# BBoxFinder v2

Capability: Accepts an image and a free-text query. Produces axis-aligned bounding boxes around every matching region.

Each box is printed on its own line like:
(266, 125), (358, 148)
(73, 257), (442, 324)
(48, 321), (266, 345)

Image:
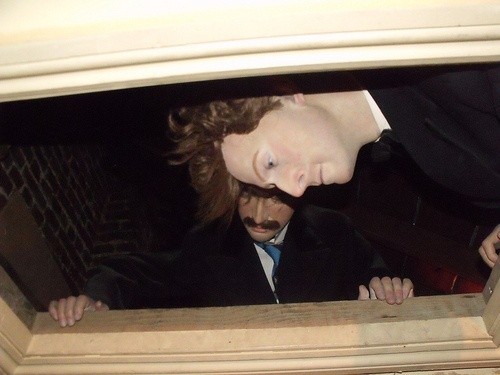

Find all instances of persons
(151, 72), (498, 272)
(47, 165), (416, 328)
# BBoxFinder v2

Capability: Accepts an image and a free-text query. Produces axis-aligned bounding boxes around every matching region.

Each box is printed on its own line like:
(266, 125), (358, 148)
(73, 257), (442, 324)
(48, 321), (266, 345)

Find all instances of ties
(257, 242), (282, 297)
